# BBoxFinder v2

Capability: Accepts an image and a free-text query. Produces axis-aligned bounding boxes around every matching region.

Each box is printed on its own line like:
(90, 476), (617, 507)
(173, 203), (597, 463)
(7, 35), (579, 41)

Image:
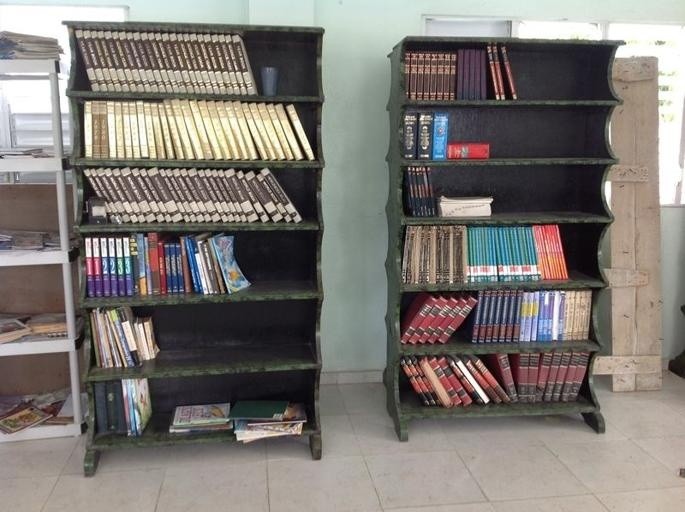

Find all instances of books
(84, 98), (315, 162)
(85, 232), (250, 296)
(402, 225), (593, 408)
(407, 165), (495, 219)
(75, 30), (259, 96)
(1, 28), (66, 62)
(449, 142), (491, 158)
(403, 113), (417, 160)
(433, 111), (448, 161)
(83, 167), (303, 224)
(94, 377), (310, 445)
(0, 144), (82, 439)
(416, 111), (433, 161)
(403, 44), (520, 102)
(88, 304), (160, 369)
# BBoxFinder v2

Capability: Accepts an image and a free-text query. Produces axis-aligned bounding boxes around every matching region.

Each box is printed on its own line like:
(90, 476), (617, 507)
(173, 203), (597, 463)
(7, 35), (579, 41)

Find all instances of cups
(260, 64), (280, 97)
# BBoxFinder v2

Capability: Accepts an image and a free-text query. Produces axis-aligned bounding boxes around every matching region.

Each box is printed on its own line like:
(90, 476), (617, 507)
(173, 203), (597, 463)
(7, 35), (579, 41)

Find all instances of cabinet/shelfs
(1, 58), (91, 443)
(383, 39), (628, 441)
(61, 20), (325, 477)
